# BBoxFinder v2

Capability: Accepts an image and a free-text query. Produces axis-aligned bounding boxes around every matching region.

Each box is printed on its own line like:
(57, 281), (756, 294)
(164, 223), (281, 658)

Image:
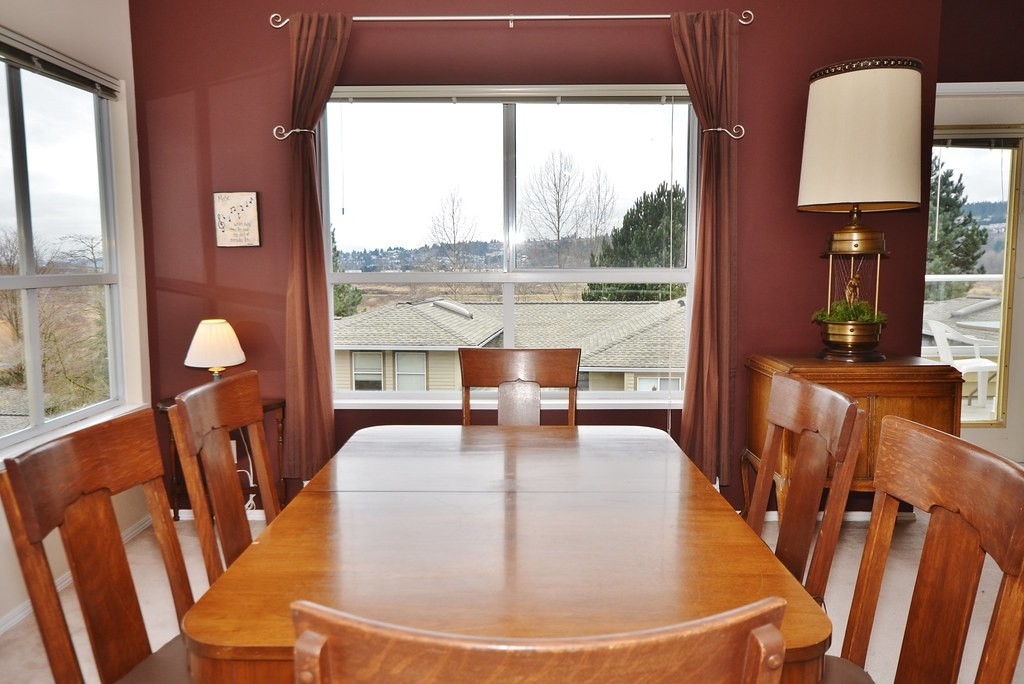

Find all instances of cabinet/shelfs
(738, 351), (964, 520)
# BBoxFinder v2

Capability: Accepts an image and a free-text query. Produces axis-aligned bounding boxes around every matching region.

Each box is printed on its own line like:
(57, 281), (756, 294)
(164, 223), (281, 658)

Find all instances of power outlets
(228, 440), (237, 465)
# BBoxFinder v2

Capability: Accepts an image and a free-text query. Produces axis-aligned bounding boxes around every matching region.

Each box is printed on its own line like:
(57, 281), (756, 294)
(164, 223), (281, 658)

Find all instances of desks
(157, 398), (286, 521)
(182, 424), (832, 684)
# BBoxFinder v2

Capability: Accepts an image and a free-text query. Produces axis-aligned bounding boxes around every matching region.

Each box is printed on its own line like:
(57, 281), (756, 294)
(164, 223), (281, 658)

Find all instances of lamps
(797, 57), (923, 363)
(183, 319), (247, 381)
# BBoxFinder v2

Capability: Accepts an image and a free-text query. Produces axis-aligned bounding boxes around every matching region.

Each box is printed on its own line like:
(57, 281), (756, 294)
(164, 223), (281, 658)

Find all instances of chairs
(927, 320), (999, 405)
(290, 596), (788, 684)
(822, 415), (1024, 684)
(0, 409), (195, 684)
(459, 347), (581, 425)
(746, 372), (867, 607)
(175, 371), (282, 588)
